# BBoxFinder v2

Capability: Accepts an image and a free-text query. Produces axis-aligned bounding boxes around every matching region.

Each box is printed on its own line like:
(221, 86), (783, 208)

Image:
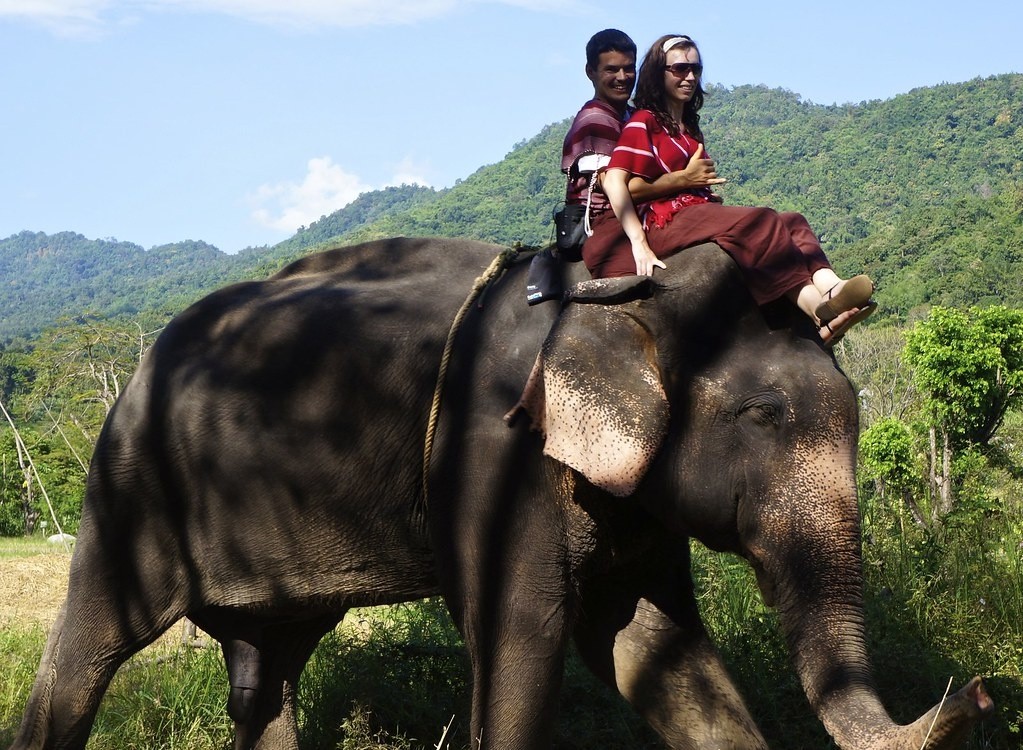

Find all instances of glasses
(665, 63), (703, 77)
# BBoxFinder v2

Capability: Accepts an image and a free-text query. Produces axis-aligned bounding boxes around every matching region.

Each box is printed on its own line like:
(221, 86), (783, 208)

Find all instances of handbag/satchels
(551, 204), (587, 262)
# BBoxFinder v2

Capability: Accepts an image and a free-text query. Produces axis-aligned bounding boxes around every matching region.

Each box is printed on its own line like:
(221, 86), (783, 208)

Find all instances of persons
(560, 29), (878, 347)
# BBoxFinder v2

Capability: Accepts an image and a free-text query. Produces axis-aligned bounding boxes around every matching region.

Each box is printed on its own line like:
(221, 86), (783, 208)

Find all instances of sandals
(815, 274), (872, 320)
(817, 303), (877, 349)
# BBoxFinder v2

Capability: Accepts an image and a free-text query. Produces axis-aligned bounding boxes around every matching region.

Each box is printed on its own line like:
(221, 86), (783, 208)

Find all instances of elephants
(12, 239), (994, 750)
